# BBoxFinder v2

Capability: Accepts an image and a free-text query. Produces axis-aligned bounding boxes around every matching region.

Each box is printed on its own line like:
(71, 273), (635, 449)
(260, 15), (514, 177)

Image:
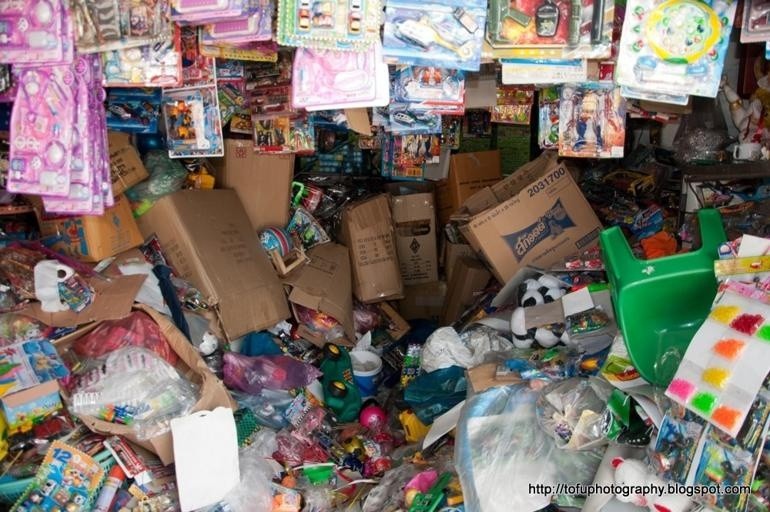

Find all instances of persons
(0, 1), (770, 512)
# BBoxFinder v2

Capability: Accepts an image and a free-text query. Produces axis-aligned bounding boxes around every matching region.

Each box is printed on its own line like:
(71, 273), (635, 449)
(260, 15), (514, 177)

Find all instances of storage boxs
(25, 127), (606, 467)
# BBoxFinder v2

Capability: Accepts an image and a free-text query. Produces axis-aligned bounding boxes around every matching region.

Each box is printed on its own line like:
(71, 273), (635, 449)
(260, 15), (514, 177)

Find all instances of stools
(598, 207), (732, 389)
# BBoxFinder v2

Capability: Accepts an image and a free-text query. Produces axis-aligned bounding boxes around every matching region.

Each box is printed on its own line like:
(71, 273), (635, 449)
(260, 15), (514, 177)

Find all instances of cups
(732, 142), (761, 162)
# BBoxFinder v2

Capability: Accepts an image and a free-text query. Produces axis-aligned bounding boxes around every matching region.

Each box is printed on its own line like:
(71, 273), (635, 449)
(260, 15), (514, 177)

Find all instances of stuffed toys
(0, 1), (770, 512)
